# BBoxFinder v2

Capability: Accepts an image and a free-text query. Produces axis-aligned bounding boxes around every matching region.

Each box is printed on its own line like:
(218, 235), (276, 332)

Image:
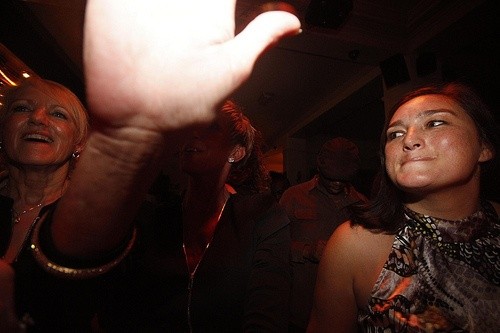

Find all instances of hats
(317, 137), (356, 182)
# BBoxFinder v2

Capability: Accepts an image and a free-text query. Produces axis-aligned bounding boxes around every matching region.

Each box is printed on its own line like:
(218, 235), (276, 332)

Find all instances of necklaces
(11, 203), (44, 223)
(183, 239), (208, 247)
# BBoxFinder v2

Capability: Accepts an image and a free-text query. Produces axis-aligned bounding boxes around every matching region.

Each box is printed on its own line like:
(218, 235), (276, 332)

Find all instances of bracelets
(30, 211), (138, 279)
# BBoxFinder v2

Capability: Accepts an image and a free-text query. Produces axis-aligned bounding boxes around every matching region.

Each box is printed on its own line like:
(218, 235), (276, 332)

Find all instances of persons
(0, 0), (500, 333)
